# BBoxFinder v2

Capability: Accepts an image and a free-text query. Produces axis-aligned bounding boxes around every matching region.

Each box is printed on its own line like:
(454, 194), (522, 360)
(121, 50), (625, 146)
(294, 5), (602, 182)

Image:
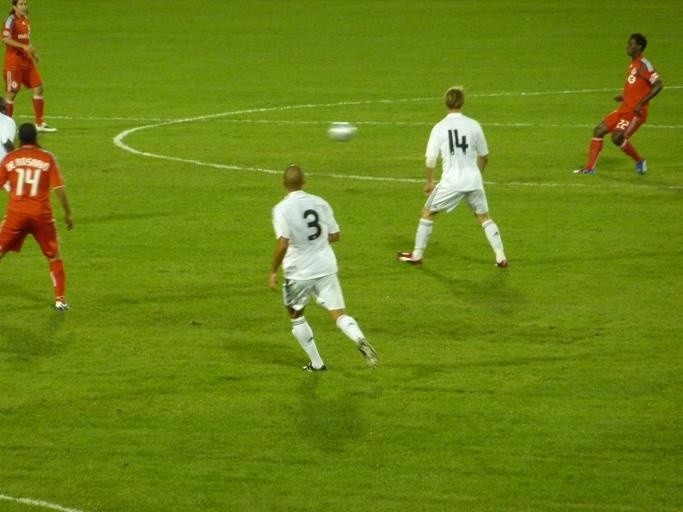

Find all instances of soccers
(330, 124), (353, 140)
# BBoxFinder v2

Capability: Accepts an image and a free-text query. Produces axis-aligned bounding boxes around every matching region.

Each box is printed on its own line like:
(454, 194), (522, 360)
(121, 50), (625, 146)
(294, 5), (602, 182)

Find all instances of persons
(0, 122), (75, 312)
(2, 0), (58, 132)
(267, 166), (378, 374)
(572, 31), (664, 177)
(397, 85), (509, 269)
(0, 96), (16, 192)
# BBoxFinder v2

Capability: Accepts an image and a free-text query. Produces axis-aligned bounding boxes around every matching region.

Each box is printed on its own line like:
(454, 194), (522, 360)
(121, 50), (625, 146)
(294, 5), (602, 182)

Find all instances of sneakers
(572, 168), (595, 175)
(52, 298), (71, 314)
(34, 122), (57, 133)
(397, 251), (424, 267)
(304, 363), (331, 373)
(495, 259), (507, 269)
(638, 159), (648, 175)
(354, 338), (380, 369)
